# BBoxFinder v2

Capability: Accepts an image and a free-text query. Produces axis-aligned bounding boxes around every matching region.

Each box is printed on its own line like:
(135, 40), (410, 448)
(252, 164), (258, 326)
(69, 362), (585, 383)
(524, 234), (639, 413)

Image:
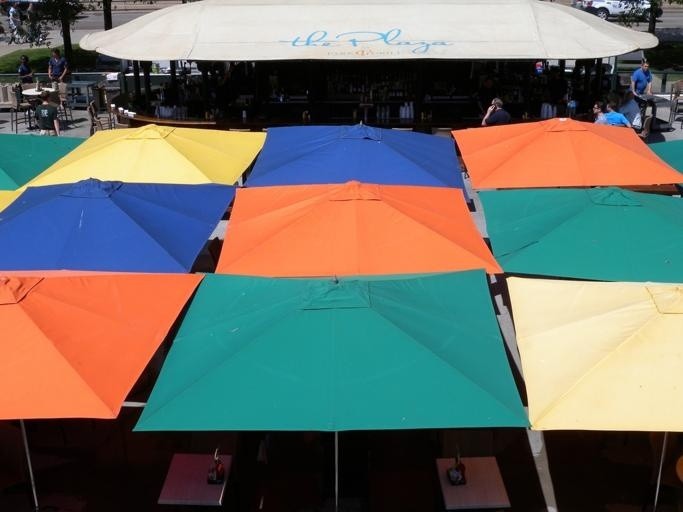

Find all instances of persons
(616, 90), (641, 130)
(630, 60), (654, 128)
(32, 91), (60, 138)
(7, 1), (22, 44)
(47, 48), (71, 109)
(479, 98), (509, 127)
(26, 3), (37, 44)
(592, 102), (607, 124)
(18, 54), (35, 84)
(605, 101), (632, 128)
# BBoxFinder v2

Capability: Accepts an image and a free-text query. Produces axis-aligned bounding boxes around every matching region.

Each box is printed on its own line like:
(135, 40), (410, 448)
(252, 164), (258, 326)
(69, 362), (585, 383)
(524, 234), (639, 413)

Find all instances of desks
(435, 456), (510, 511)
(157, 451), (233, 507)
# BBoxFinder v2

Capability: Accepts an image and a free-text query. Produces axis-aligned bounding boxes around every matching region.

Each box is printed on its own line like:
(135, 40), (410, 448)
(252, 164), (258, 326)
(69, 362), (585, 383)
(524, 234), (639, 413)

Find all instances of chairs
(635, 114), (653, 144)
(8, 81), (73, 134)
(87, 100), (115, 131)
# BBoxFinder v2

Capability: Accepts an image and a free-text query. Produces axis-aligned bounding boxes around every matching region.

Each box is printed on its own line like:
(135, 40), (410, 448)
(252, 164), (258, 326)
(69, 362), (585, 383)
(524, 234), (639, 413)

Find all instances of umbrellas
(644, 141), (682, 174)
(448, 117), (682, 190)
(0, 191), (21, 212)
(19, 121), (266, 186)
(213, 178), (503, 277)
(501, 276), (683, 510)
(476, 188), (682, 283)
(0, 268), (205, 512)
(0, 132), (86, 190)
(240, 121), (471, 204)
(75, 1), (665, 64)
(131, 271), (532, 512)
(0, 181), (234, 273)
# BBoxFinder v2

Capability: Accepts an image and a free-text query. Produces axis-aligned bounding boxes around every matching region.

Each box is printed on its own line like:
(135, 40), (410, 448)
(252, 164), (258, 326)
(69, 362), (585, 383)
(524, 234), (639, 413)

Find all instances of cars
(575, 0), (663, 21)
